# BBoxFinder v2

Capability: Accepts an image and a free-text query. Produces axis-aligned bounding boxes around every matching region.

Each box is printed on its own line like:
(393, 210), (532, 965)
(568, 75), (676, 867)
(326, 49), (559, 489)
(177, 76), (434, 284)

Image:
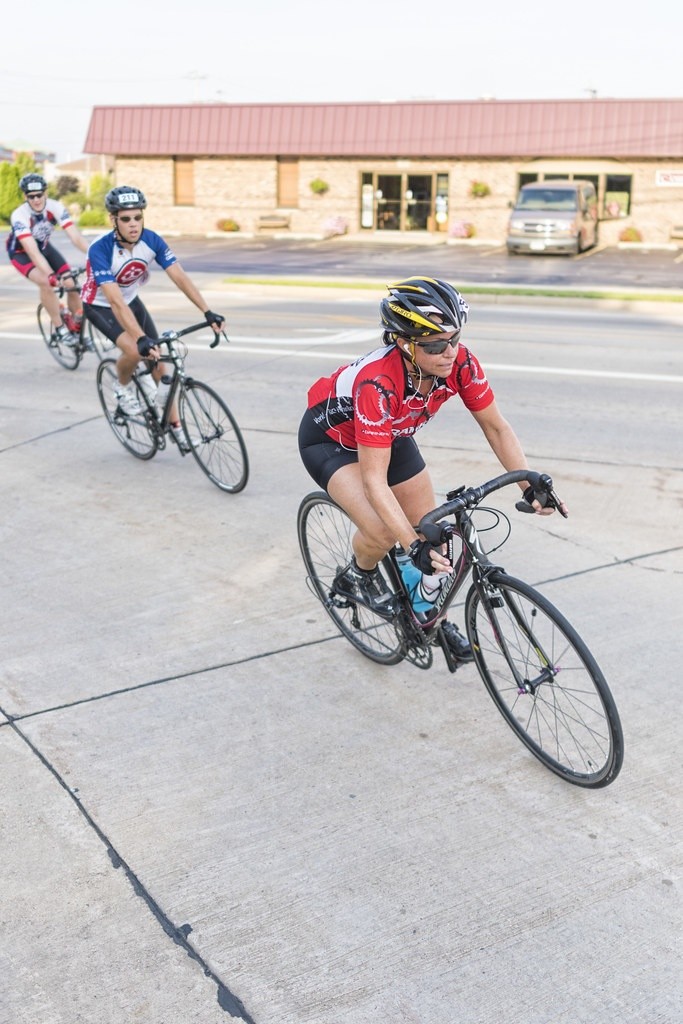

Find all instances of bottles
(394, 542), (435, 612)
(136, 364), (158, 402)
(155, 375), (172, 404)
(73, 308), (82, 332)
(417, 571), (446, 603)
(60, 304), (74, 331)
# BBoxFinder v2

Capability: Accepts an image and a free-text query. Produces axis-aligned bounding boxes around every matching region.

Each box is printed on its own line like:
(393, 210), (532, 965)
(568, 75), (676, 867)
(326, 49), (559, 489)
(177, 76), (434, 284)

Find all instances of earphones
(404, 343), (413, 356)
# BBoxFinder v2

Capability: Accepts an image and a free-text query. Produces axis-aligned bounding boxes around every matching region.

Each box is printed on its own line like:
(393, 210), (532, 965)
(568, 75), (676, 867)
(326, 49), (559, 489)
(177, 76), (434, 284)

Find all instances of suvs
(506, 179), (598, 255)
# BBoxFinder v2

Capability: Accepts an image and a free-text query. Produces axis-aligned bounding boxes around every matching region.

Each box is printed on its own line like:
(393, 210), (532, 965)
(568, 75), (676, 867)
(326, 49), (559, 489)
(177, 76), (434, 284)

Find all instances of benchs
(258, 214), (292, 230)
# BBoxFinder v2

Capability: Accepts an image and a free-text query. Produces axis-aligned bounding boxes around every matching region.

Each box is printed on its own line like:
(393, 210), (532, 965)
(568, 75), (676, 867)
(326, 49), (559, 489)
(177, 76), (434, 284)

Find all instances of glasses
(113, 214), (144, 221)
(398, 331), (461, 354)
(25, 192), (46, 199)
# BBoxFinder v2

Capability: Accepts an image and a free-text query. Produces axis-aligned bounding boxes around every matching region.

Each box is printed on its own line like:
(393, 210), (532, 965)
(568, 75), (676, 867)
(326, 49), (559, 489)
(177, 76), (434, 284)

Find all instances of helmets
(379, 276), (470, 336)
(105, 186), (147, 211)
(20, 174), (47, 191)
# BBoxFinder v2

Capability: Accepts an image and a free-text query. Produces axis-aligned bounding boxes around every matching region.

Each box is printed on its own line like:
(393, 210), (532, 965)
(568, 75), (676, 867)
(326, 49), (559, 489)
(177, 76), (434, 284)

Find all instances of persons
(5, 174), (91, 346)
(298, 275), (569, 660)
(80, 186), (224, 448)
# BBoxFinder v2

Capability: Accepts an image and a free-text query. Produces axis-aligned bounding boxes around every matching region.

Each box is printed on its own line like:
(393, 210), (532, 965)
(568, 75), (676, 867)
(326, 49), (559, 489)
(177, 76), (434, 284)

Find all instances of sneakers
(168, 426), (195, 450)
(434, 621), (478, 661)
(350, 554), (401, 618)
(55, 323), (79, 346)
(112, 382), (142, 415)
(84, 336), (95, 352)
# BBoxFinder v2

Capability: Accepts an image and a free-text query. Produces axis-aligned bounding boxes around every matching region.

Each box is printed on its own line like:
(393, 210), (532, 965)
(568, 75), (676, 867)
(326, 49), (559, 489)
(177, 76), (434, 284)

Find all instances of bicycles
(297, 470), (627, 792)
(96, 321), (250, 494)
(38, 268), (120, 371)
